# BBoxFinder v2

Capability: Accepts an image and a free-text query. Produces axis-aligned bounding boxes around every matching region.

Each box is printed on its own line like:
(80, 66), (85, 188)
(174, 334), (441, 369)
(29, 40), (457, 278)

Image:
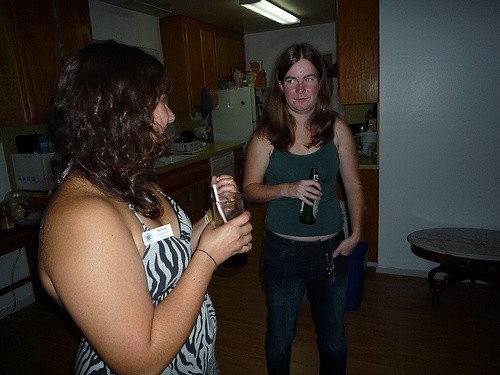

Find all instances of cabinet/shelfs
(336, 161), (379, 264)
(335, 0), (379, 105)
(0, 0), (101, 128)
(0, 209), (46, 323)
(160, 15), (245, 115)
(153, 138), (247, 224)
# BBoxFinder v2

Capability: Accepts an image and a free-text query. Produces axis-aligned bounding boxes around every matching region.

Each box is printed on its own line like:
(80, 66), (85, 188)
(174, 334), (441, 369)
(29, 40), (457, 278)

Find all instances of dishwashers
(210, 151), (235, 183)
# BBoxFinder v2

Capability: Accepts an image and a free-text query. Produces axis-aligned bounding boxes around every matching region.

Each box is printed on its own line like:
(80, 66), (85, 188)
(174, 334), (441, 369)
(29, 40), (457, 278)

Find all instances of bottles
(0, 202), (17, 233)
(260, 72), (267, 88)
(299, 167), (320, 225)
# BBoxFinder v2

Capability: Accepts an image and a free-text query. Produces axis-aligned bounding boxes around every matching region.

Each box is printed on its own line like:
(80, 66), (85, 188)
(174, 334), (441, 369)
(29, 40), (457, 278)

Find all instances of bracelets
(196, 249), (218, 271)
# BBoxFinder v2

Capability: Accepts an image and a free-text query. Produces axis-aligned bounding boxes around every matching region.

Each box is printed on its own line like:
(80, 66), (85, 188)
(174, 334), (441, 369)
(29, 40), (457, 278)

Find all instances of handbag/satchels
(333, 239), (369, 311)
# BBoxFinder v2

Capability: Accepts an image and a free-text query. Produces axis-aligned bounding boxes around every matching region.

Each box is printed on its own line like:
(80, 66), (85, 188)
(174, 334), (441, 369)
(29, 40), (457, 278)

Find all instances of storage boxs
(11, 150), (63, 191)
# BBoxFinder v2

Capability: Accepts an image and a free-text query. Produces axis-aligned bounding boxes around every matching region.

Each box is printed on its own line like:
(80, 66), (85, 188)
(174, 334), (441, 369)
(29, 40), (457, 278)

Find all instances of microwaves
(10, 151), (65, 191)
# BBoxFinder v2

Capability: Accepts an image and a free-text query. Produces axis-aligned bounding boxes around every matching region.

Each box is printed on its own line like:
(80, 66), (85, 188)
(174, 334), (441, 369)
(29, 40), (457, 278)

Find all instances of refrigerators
(210, 87), (272, 153)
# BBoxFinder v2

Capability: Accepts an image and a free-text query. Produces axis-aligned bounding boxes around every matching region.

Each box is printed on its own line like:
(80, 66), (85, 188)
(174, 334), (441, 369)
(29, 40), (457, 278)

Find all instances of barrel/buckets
(361, 132), (377, 154)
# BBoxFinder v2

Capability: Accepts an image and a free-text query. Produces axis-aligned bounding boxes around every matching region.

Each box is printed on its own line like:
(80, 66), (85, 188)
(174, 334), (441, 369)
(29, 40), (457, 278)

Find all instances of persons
(38, 39), (252, 375)
(243, 42), (365, 375)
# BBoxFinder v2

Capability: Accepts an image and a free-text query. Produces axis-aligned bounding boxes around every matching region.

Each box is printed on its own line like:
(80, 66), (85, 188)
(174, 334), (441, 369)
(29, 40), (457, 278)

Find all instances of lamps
(239, 0), (301, 25)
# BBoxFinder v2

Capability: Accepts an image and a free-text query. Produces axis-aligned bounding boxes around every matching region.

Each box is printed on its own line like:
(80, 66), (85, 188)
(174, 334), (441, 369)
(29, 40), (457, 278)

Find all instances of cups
(210, 191), (248, 269)
(11, 205), (26, 221)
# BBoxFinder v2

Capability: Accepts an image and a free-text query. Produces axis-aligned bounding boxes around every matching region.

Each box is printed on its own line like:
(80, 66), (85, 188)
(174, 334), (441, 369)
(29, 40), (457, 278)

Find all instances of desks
(407, 228), (500, 313)
